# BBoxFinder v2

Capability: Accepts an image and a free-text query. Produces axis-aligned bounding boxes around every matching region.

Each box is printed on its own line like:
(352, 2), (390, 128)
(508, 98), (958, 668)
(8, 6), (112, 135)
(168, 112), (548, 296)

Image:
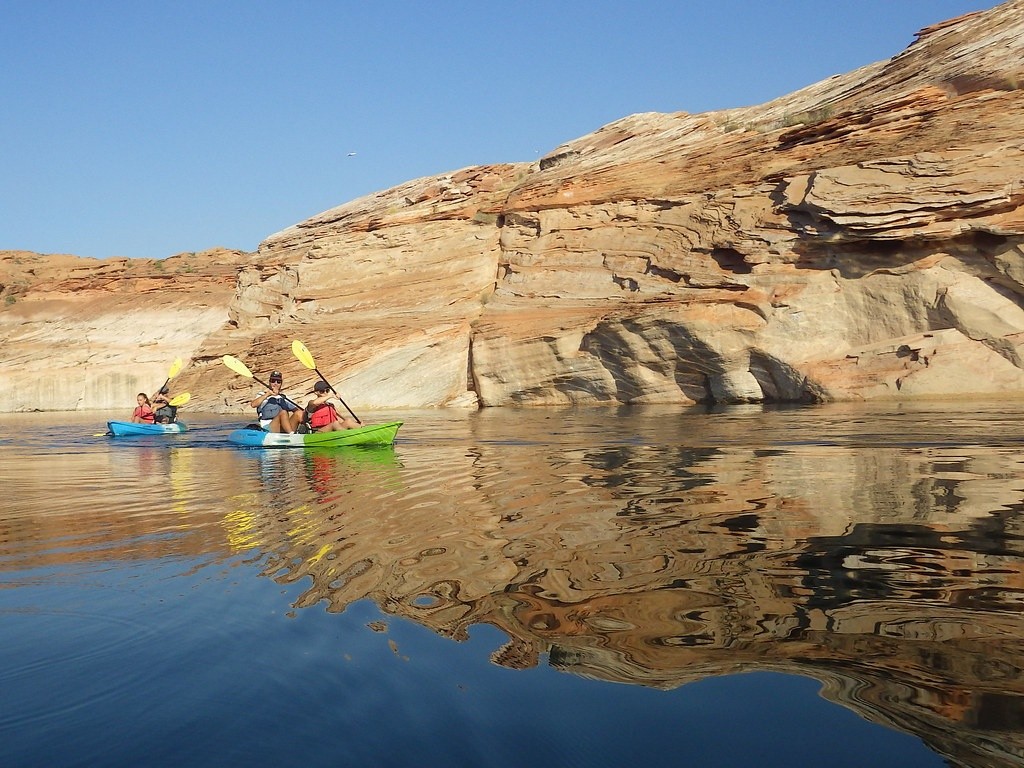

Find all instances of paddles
(152, 356), (184, 405)
(291, 339), (362, 426)
(221, 354), (306, 414)
(92, 392), (191, 438)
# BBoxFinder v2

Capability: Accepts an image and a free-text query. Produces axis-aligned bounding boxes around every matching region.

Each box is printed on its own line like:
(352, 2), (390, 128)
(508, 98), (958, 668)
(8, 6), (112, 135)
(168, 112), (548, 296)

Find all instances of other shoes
(299, 424), (308, 434)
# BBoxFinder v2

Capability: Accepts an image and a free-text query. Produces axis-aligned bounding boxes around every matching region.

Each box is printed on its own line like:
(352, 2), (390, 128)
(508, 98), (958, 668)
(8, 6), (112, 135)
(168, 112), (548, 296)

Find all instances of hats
(160, 386), (169, 392)
(270, 371), (282, 380)
(314, 381), (329, 391)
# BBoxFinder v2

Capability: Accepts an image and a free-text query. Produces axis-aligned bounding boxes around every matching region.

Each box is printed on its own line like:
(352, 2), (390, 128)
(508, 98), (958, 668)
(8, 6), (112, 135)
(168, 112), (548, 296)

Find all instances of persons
(251, 371), (312, 434)
(150, 387), (177, 424)
(129, 393), (155, 424)
(305, 381), (364, 433)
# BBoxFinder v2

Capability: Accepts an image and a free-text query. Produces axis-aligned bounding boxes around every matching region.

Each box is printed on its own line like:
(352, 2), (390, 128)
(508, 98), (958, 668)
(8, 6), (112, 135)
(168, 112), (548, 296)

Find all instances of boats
(106, 419), (190, 442)
(225, 420), (405, 452)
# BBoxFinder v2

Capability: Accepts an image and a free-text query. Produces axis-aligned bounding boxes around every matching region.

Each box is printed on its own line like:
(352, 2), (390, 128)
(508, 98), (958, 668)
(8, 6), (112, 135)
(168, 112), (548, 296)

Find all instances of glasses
(269, 379), (281, 384)
(317, 389), (330, 394)
(162, 391), (169, 394)
